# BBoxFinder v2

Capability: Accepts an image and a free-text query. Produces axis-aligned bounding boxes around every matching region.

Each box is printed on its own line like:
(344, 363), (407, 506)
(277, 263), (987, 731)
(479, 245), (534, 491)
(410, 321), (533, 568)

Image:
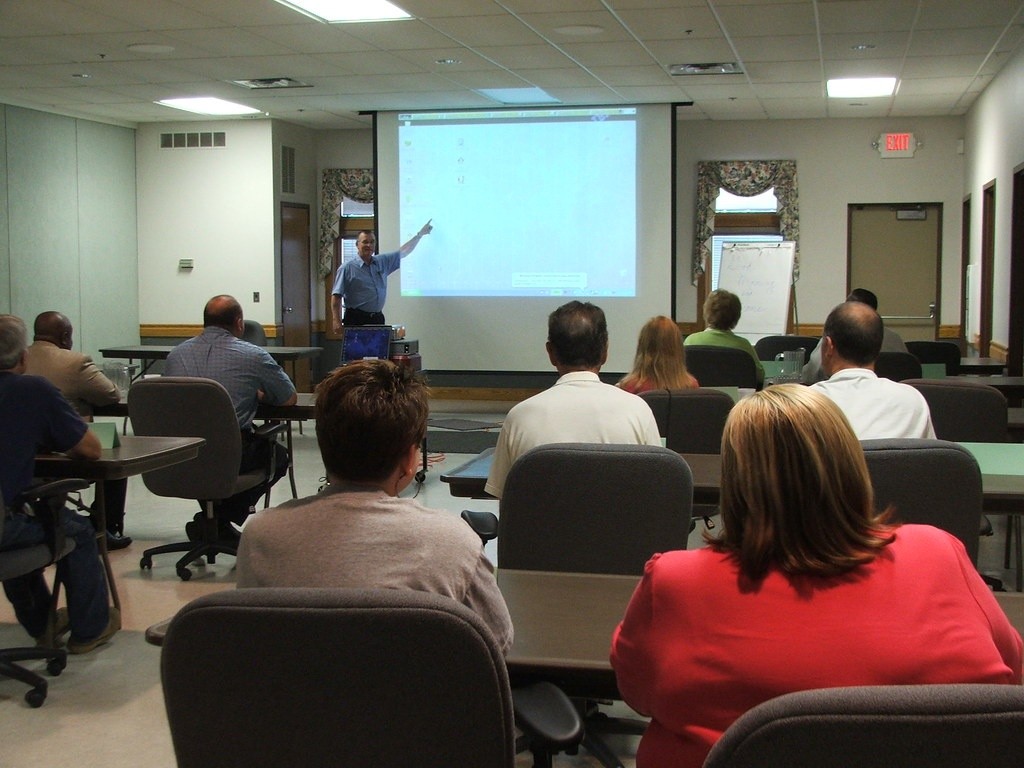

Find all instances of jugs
(775, 348), (805, 382)
(101, 364), (130, 399)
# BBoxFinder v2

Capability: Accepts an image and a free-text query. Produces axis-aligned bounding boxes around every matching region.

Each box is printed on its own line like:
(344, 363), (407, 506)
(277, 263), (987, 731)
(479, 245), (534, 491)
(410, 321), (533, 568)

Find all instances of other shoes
(185, 512), (243, 545)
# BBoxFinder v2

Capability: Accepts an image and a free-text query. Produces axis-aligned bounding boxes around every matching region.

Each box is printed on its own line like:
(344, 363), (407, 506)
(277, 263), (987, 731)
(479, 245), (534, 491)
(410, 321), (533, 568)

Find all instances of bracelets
(417, 232), (422, 238)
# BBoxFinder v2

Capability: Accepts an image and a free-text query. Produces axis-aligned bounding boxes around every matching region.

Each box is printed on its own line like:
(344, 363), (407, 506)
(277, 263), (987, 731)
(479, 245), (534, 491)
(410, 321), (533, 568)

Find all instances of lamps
(871, 132), (922, 158)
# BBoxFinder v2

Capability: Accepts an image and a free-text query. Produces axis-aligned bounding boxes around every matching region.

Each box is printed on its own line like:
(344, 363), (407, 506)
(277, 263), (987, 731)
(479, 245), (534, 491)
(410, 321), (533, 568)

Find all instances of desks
(36, 344), (324, 652)
(147, 355), (1023, 701)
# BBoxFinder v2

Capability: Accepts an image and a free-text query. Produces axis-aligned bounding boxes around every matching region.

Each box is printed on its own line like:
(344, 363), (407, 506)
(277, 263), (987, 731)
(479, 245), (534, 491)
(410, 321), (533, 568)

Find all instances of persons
(685, 288), (766, 382)
(331, 219), (434, 336)
(806, 303), (940, 441)
(787, 286), (910, 385)
(18, 310), (133, 553)
(609, 384), (1024, 768)
(484, 300), (664, 513)
(234, 359), (516, 658)
(0, 313), (122, 654)
(160, 295), (297, 555)
(615, 314), (700, 396)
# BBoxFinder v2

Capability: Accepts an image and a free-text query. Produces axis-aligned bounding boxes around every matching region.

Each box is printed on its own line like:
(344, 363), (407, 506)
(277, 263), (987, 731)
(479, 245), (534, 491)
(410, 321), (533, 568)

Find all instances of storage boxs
(389, 338), (419, 355)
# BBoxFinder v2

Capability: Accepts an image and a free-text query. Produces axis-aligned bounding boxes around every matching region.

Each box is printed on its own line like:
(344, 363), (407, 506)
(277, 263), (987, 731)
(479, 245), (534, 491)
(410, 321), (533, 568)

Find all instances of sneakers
(106, 529), (132, 549)
(66, 607), (122, 654)
(35, 607), (70, 644)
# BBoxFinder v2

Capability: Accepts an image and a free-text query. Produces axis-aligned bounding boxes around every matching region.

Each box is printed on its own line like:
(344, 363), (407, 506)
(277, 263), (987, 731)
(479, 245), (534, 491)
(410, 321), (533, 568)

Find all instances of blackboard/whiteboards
(716, 241), (797, 336)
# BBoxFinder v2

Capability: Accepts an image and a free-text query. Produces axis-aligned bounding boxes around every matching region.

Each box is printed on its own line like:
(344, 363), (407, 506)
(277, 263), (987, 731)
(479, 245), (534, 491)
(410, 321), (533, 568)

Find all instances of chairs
(701, 685), (1024, 768)
(461, 441), (693, 768)
(637, 389), (736, 529)
(899, 379), (1008, 591)
(160, 587), (582, 768)
(873, 352), (922, 382)
(240, 320), (268, 347)
(127, 377), (291, 580)
(904, 340), (961, 356)
(0, 476), (89, 709)
(754, 335), (820, 361)
(683, 345), (756, 389)
(856, 438), (983, 574)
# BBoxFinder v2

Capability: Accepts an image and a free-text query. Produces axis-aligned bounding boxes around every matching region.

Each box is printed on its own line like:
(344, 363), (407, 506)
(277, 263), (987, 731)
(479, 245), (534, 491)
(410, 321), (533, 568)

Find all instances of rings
(334, 332), (337, 335)
